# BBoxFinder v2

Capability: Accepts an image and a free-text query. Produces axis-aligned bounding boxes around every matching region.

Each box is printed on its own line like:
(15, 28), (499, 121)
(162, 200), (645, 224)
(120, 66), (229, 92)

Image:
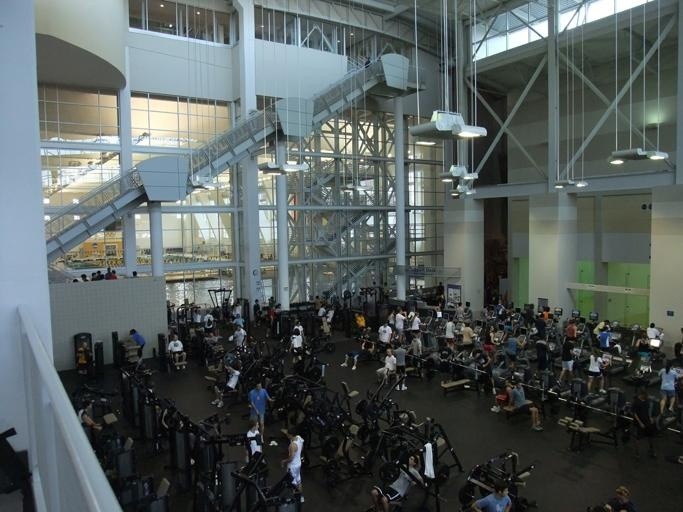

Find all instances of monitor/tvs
(427, 301), (661, 407)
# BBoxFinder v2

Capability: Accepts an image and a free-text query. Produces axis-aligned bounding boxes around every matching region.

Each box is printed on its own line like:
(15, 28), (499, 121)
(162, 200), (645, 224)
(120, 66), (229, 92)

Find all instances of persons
(370, 455), (422, 511)
(133, 271), (137, 277)
(471, 481), (512, 512)
(340, 282), (682, 430)
(73, 267), (119, 282)
(246, 381), (305, 503)
(588, 486), (634, 511)
(71, 281), (334, 406)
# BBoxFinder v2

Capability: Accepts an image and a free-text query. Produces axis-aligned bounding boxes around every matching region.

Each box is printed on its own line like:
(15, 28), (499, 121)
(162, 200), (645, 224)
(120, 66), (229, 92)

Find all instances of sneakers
(600, 389), (606, 394)
(210, 398), (224, 408)
(341, 363), (357, 370)
(491, 405), (499, 413)
(395, 387), (408, 391)
(177, 365), (186, 370)
(532, 425), (544, 432)
(668, 407), (674, 413)
(300, 496), (305, 503)
(556, 380), (563, 388)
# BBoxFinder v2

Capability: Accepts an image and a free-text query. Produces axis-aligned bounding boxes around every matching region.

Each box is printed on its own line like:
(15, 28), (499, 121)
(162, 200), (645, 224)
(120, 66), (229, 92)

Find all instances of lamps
(553, 0), (590, 190)
(188, 0), (230, 192)
(605, 1), (670, 165)
(254, 0), (310, 179)
(407, 1), (489, 198)
